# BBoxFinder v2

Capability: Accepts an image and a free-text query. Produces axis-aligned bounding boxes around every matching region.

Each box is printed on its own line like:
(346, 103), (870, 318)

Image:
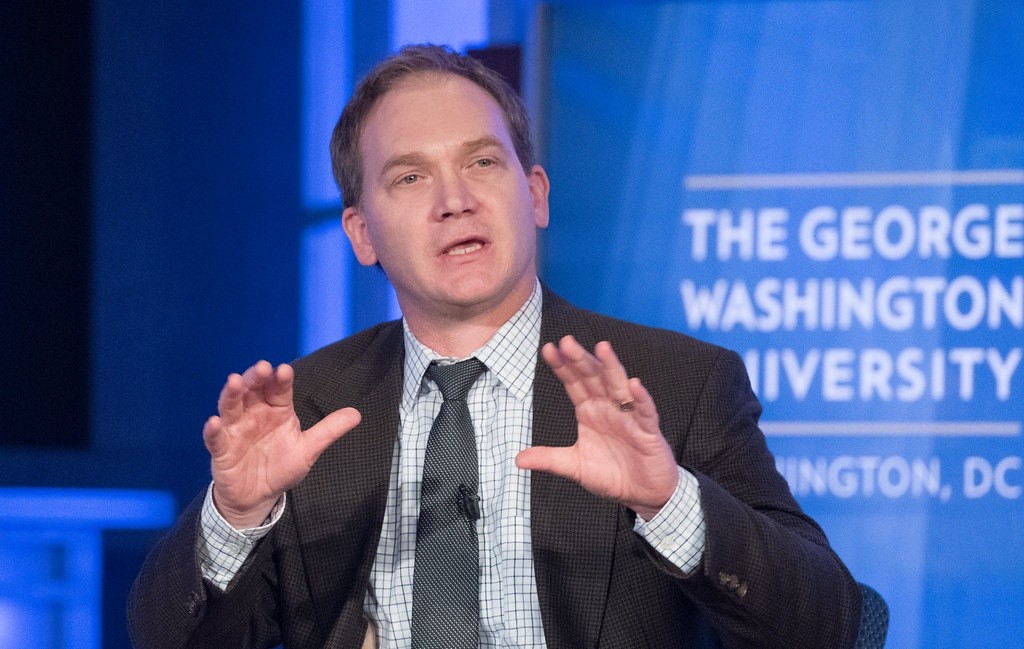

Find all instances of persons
(124, 44), (889, 648)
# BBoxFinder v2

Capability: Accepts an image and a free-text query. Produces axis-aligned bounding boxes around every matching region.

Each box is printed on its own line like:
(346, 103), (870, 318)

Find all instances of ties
(411, 359), (489, 648)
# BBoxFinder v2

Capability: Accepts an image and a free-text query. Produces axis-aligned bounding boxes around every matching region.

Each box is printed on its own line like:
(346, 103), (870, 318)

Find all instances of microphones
(458, 485), (478, 520)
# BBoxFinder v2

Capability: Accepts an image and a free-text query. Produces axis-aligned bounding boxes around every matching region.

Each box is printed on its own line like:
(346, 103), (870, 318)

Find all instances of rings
(614, 398), (635, 412)
